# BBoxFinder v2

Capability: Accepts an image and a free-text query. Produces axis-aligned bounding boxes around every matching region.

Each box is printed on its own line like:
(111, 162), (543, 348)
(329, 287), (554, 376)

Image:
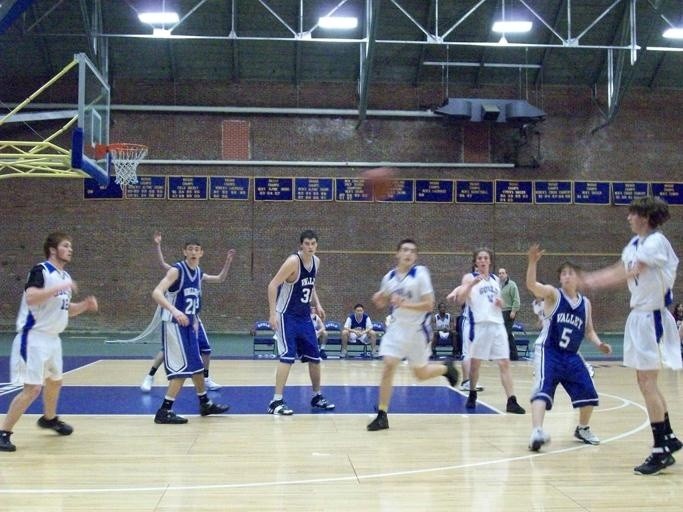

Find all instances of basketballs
(364, 167), (400, 198)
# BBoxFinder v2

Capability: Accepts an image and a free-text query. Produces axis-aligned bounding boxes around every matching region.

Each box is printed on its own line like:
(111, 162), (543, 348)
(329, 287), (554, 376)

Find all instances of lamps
(490, 14), (534, 35)
(136, 7), (182, 27)
(661, 22), (682, 40)
(316, 8), (359, 31)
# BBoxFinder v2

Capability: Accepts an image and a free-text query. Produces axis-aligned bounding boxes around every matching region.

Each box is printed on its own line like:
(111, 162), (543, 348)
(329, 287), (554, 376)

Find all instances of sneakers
(367, 410), (389, 430)
(267, 399), (293, 415)
(528, 430), (550, 451)
(574, 426), (600, 445)
(319, 349), (328, 359)
(465, 390), (477, 409)
(340, 351), (348, 359)
(154, 409), (188, 424)
(142, 375), (154, 391)
(0, 430), (16, 452)
(204, 378), (222, 391)
(453, 348), (460, 358)
(371, 352), (379, 359)
(665, 437), (682, 453)
(311, 395), (335, 410)
(459, 378), (483, 391)
(506, 395), (525, 413)
(633, 451), (675, 475)
(443, 360), (459, 386)
(37, 415), (75, 435)
(200, 402), (229, 416)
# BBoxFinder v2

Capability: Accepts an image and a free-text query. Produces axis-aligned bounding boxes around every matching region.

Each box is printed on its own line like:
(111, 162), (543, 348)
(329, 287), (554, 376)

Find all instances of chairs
(252, 319), (531, 361)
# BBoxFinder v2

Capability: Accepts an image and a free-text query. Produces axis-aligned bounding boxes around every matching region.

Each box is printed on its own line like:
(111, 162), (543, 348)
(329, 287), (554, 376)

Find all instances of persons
(267, 229), (335, 415)
(143, 230), (236, 424)
(340, 304), (379, 359)
(310, 306), (328, 359)
(447, 247), (526, 414)
(673, 303), (683, 345)
(422, 302), (463, 359)
(578, 194), (683, 475)
(367, 240), (460, 431)
(0, 232), (98, 451)
(526, 242), (612, 452)
